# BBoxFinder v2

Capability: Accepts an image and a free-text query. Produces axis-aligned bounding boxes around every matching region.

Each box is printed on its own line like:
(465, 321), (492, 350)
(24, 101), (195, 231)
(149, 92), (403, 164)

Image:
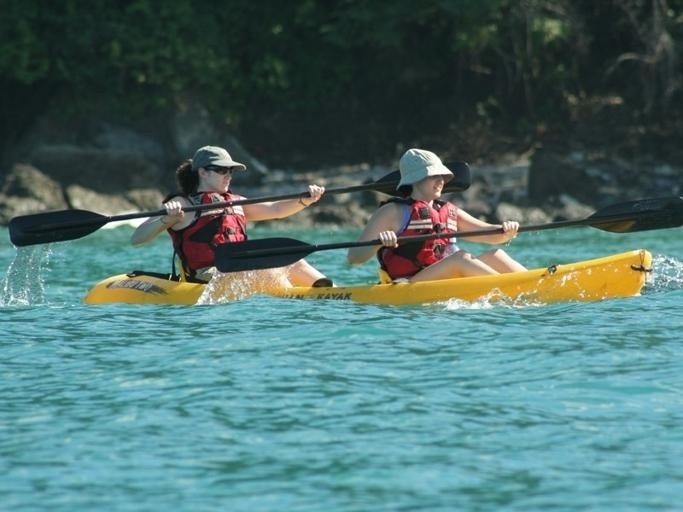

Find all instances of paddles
(9, 161), (470, 246)
(214, 197), (683, 272)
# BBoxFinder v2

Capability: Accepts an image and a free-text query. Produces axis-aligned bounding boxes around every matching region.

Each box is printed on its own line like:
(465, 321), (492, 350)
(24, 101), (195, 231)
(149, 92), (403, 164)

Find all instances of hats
(395, 148), (453, 192)
(191, 145), (246, 176)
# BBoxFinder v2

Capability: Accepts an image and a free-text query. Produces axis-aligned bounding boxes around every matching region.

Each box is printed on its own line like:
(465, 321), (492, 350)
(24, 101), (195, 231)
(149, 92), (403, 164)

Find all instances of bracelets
(159, 216), (166, 224)
(297, 197), (306, 206)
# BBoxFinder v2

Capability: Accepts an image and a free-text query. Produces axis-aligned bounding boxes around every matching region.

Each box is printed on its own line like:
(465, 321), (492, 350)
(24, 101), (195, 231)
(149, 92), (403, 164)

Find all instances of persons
(129, 145), (337, 289)
(348, 149), (528, 286)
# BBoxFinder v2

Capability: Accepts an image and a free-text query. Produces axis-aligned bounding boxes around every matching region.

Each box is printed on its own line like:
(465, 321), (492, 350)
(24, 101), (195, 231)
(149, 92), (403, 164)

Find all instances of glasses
(204, 165), (232, 175)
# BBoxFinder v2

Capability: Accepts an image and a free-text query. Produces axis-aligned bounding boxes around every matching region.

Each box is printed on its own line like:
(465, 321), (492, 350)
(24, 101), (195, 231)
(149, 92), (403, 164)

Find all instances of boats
(83, 249), (652, 305)
